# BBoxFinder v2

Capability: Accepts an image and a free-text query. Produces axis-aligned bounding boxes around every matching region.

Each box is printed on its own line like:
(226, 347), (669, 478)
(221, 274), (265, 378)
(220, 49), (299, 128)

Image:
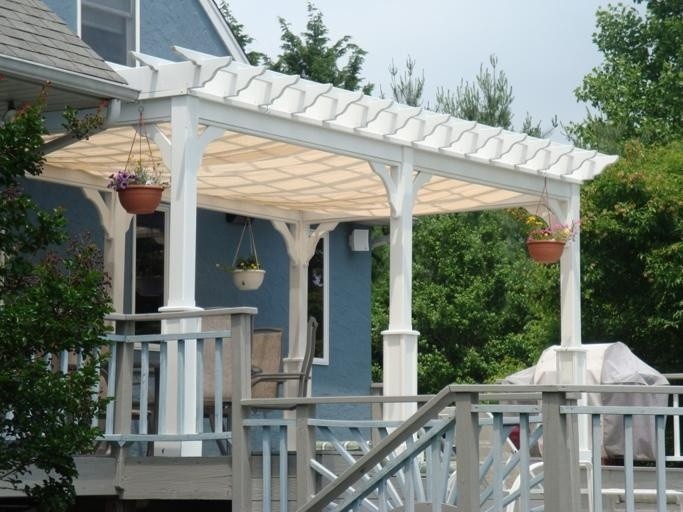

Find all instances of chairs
(35, 307), (319, 457)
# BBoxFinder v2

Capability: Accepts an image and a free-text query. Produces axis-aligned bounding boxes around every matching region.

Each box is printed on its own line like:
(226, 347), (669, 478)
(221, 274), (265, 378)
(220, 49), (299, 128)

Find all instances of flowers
(104, 170), (171, 193)
(231, 255), (262, 269)
(522, 211), (583, 243)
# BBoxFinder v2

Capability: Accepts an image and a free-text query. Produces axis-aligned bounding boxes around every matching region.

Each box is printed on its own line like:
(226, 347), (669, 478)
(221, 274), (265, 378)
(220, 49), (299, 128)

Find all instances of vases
(527, 240), (566, 262)
(116, 185), (164, 215)
(133, 274), (165, 298)
(230, 268), (266, 292)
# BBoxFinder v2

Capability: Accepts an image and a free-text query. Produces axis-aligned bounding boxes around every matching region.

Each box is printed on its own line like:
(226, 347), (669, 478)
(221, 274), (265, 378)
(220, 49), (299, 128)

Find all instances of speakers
(349, 229), (369, 252)
